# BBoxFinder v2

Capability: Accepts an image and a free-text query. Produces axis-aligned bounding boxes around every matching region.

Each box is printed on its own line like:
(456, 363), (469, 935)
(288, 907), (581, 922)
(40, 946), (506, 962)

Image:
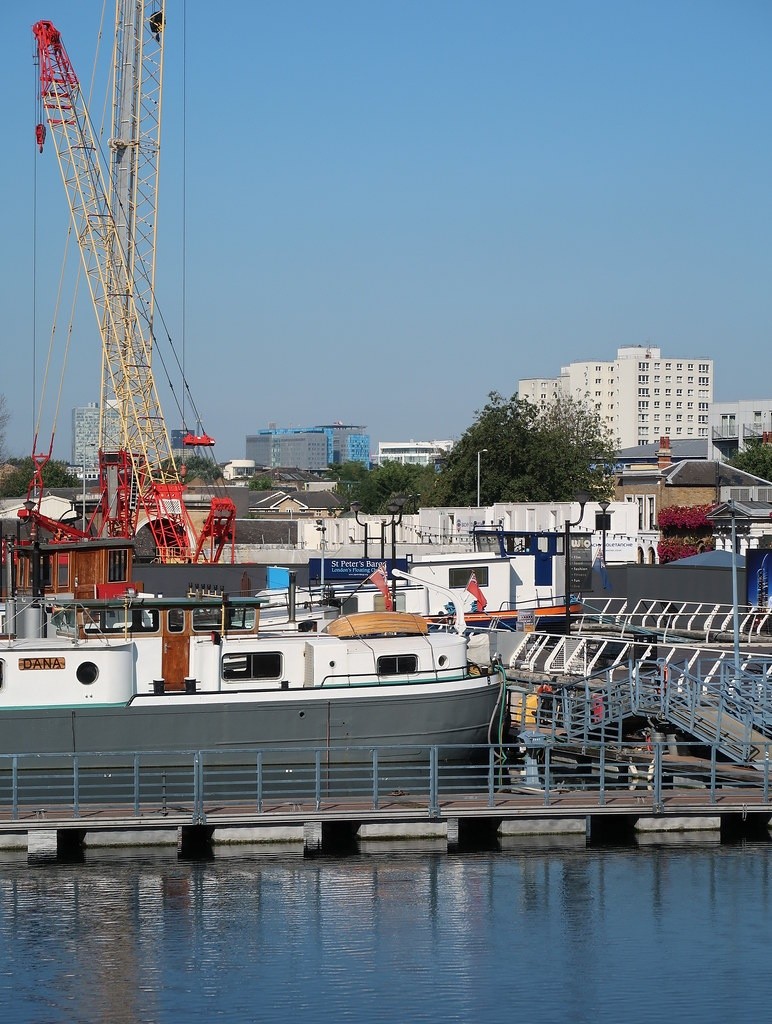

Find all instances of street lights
(477, 448), (488, 507)
(380, 501), (400, 559)
(726, 496), (742, 687)
(564, 486), (593, 639)
(598, 495), (611, 563)
(16, 500), (37, 546)
(316, 526), (327, 599)
(391, 493), (409, 612)
(349, 500), (367, 558)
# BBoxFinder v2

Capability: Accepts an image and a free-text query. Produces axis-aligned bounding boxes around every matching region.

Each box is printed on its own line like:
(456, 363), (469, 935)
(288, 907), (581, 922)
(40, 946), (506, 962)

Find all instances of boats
(2, 500), (771, 806)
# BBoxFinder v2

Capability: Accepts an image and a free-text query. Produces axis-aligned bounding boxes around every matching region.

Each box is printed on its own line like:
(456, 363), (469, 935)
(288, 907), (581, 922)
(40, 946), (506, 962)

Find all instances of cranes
(16, 0), (237, 567)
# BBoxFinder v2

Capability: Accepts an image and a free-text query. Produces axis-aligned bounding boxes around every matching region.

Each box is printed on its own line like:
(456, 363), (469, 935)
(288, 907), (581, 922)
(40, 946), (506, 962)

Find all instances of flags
(371, 562), (393, 612)
(593, 550), (614, 592)
(468, 574), (488, 612)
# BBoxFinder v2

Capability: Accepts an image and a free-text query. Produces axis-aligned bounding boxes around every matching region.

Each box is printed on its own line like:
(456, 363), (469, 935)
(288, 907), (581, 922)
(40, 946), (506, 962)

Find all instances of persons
(767, 596), (772, 635)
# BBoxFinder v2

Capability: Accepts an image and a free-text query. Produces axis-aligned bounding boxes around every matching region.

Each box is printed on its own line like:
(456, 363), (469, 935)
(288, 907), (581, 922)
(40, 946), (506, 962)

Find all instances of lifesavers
(647, 758), (655, 791)
(627, 766), (638, 790)
(537, 685), (551, 705)
